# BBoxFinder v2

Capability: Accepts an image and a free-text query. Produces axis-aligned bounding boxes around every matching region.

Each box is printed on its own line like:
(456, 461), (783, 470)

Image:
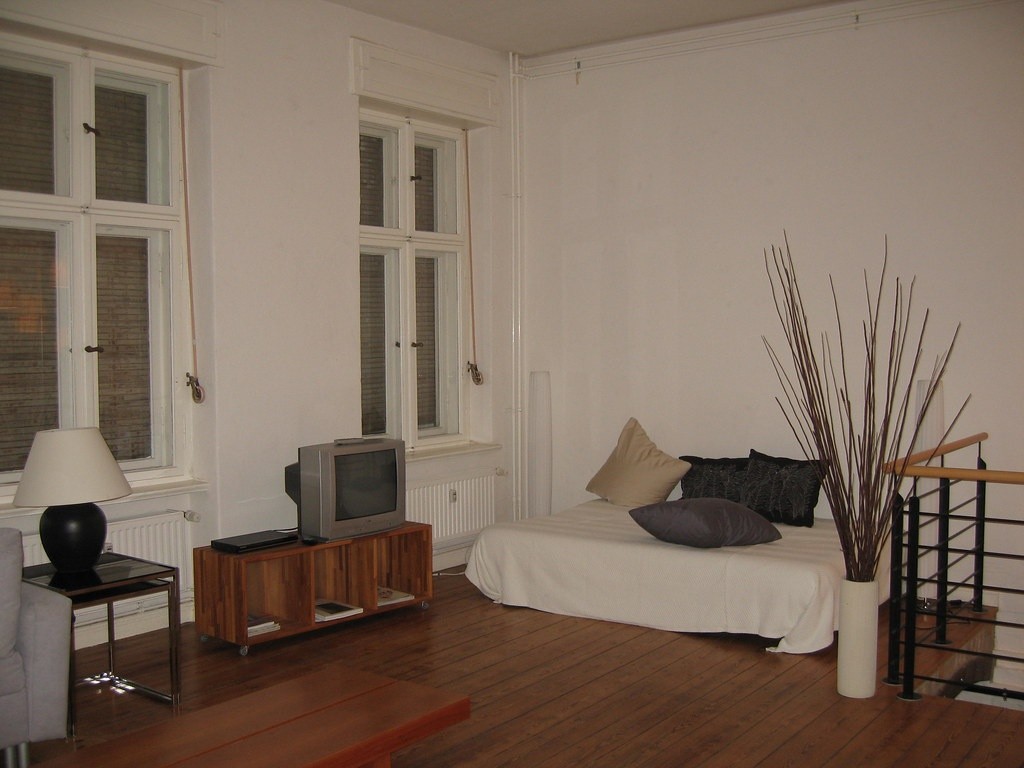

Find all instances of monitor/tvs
(284, 437), (406, 543)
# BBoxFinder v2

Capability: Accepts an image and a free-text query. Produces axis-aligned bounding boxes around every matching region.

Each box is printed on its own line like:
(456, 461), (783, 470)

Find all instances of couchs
(0, 527), (73, 768)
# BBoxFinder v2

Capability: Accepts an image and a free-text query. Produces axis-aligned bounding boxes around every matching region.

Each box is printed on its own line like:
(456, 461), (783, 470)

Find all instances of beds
(464, 499), (908, 654)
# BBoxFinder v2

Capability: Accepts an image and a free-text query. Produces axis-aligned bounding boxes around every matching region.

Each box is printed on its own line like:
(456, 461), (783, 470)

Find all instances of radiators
(405, 473), (497, 544)
(22, 508), (201, 617)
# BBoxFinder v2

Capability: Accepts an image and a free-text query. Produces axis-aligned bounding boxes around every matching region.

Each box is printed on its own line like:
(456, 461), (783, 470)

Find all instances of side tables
(22, 552), (182, 743)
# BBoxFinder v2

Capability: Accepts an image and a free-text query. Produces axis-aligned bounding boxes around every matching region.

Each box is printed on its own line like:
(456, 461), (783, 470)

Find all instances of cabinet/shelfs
(193, 521), (433, 656)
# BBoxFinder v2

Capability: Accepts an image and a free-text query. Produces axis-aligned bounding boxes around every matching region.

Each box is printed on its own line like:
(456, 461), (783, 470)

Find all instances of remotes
(334, 438), (364, 445)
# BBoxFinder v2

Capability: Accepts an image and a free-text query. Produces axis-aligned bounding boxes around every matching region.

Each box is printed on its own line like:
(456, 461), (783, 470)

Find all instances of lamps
(12, 426), (133, 575)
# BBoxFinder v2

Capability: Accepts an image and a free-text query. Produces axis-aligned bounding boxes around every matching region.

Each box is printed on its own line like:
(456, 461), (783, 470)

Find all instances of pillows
(585, 417), (692, 508)
(679, 456), (749, 504)
(628, 497), (783, 549)
(739, 449), (831, 528)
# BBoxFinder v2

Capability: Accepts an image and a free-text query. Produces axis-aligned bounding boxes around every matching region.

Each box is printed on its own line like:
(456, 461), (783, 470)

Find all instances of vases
(836, 575), (879, 698)
